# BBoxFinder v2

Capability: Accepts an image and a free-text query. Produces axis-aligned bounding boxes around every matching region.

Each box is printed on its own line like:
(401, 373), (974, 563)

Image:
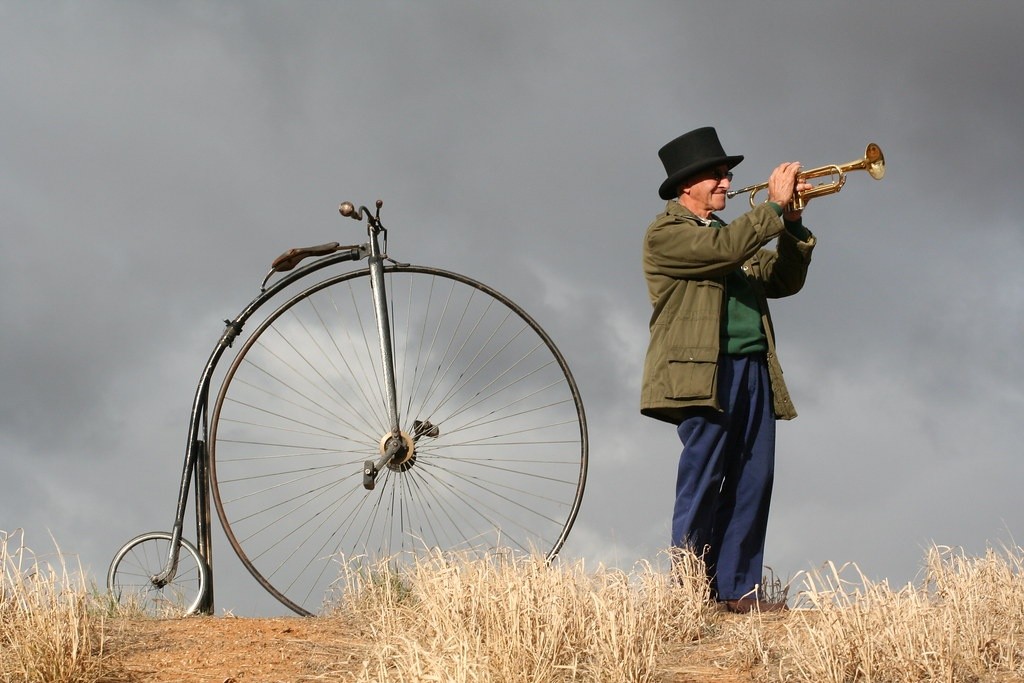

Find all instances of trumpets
(726, 142), (886, 213)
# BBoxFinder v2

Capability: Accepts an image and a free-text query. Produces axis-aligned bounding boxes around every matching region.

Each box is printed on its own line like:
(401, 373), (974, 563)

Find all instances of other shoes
(726, 599), (780, 613)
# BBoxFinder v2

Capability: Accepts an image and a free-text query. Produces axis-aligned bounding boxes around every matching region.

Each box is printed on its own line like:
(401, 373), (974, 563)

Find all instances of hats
(658, 127), (744, 201)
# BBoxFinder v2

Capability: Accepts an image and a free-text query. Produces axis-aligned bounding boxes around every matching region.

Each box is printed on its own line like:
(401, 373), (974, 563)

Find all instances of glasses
(687, 168), (733, 182)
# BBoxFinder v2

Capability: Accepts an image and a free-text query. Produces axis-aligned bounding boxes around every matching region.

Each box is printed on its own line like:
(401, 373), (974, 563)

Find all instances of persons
(640, 125), (817, 616)
